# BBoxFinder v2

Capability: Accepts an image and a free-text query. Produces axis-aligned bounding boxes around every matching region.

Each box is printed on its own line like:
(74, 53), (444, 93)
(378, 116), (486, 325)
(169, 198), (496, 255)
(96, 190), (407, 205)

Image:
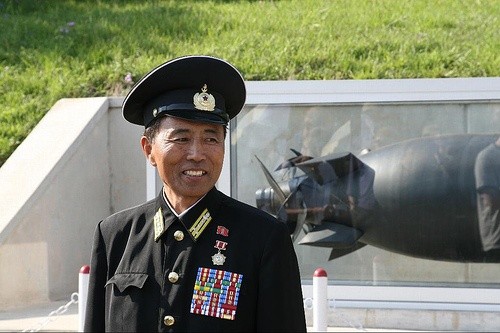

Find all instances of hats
(121, 54), (247, 128)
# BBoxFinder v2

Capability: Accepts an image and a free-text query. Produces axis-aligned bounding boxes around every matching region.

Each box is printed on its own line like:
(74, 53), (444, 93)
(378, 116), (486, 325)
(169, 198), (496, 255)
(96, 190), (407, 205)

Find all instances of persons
(474, 136), (500, 266)
(84, 54), (307, 333)
(287, 106), (457, 157)
